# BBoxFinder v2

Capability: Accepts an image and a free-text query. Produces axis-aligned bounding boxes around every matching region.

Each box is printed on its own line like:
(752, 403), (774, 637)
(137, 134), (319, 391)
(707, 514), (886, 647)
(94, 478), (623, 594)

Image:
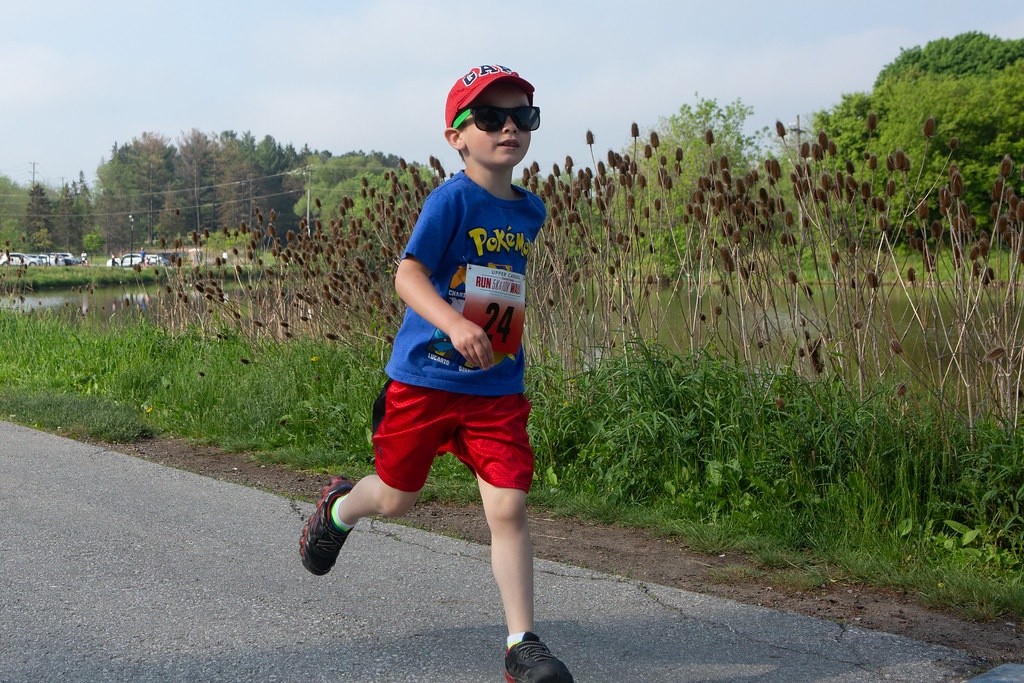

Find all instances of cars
(106, 253), (170, 267)
(0, 252), (82, 267)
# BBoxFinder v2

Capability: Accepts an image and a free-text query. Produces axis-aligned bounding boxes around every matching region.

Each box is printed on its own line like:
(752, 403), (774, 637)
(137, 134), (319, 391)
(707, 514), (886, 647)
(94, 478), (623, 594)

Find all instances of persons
(111, 252), (115, 266)
(81, 251), (88, 267)
(297, 65), (573, 683)
(121, 255), (124, 266)
(140, 247), (145, 266)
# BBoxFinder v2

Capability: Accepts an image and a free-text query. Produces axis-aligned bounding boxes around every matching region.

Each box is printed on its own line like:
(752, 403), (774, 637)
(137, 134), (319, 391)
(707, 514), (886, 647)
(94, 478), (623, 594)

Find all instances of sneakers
(300, 477), (355, 575)
(504, 632), (573, 683)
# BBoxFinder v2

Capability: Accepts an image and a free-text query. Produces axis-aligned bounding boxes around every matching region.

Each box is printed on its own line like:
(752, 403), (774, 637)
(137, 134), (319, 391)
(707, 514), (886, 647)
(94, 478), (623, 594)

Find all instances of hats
(445, 65), (536, 128)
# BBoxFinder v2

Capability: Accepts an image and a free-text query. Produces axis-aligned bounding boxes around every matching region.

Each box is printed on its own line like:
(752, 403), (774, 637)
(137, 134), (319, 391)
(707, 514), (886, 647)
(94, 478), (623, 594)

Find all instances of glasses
(453, 106), (542, 131)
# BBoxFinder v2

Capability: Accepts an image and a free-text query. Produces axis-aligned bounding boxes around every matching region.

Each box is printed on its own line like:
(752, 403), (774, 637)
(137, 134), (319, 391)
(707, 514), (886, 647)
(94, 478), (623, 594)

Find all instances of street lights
(128, 214), (135, 267)
